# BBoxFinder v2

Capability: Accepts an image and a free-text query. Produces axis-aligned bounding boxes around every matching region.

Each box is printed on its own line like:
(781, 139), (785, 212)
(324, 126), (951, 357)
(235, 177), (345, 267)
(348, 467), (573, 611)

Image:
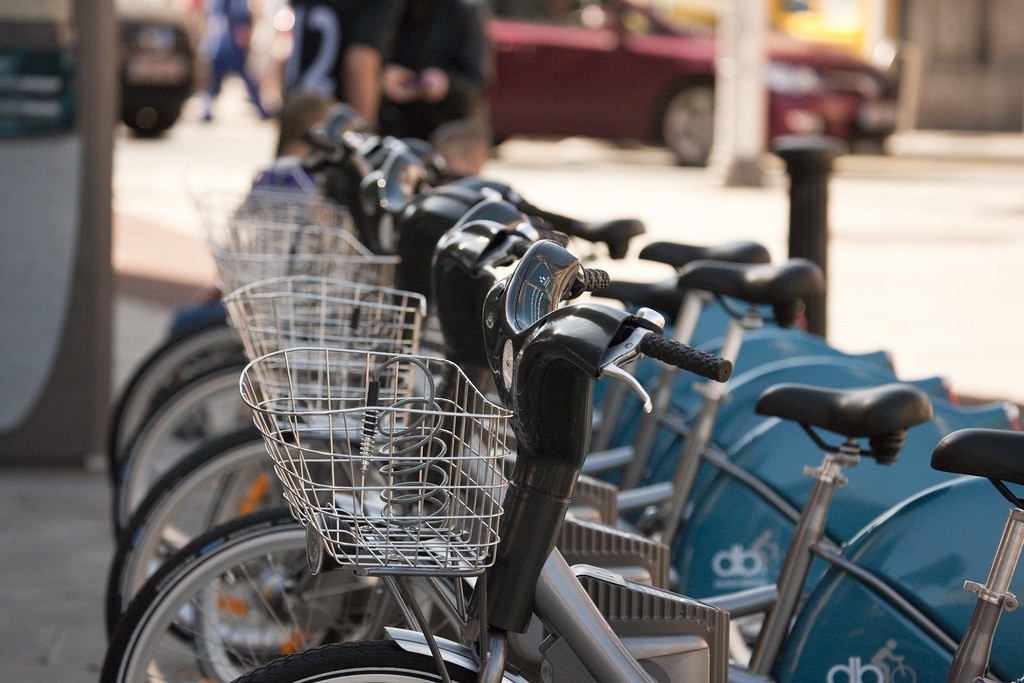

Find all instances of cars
(1, 2), (201, 140)
(484, 0), (903, 165)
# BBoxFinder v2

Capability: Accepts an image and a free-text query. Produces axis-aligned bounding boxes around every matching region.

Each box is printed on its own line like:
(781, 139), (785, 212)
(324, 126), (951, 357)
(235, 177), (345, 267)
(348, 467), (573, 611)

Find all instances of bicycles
(97, 112), (1024, 683)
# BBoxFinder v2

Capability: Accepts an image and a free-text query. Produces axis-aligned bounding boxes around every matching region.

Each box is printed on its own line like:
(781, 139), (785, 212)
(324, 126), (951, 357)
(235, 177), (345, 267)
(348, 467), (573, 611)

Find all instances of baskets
(224, 217), (375, 366)
(236, 186), (353, 275)
(240, 343), (512, 575)
(222, 273), (428, 446)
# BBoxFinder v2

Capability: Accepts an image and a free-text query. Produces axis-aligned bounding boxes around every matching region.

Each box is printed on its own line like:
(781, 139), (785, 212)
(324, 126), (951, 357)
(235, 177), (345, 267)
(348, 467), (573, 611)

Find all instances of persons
(276, 0), (490, 183)
(198, 0), (267, 120)
